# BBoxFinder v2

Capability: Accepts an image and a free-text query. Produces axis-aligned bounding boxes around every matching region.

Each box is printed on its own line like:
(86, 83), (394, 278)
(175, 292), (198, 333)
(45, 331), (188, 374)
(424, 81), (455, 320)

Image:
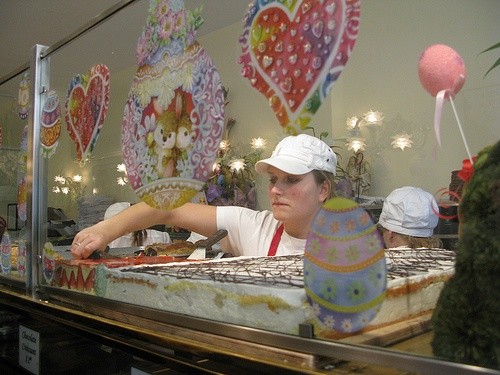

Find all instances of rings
(74, 243), (81, 246)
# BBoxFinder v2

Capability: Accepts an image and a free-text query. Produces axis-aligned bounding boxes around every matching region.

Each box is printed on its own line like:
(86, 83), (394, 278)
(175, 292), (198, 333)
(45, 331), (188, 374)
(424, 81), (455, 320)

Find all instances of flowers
(200, 137), (268, 210)
(303, 109), (414, 203)
(51, 174), (99, 206)
(116, 162), (129, 186)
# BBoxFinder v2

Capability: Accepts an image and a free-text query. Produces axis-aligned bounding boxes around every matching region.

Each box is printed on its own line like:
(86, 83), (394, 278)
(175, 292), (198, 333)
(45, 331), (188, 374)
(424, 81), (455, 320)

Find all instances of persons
(69, 134), (337, 260)
(103, 202), (172, 248)
(373, 186), (443, 250)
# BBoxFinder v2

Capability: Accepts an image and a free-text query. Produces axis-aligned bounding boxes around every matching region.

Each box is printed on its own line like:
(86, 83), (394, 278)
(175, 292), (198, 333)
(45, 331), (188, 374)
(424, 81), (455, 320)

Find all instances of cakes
(44, 248), (456, 340)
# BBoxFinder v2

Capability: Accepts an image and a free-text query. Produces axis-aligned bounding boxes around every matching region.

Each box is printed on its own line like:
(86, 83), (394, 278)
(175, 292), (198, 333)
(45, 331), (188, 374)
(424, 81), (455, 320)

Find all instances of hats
(254, 133), (339, 178)
(378, 186), (440, 239)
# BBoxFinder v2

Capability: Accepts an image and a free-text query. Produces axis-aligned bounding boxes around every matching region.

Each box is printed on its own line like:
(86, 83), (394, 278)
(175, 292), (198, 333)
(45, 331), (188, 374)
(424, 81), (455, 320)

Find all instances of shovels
(186, 229), (230, 262)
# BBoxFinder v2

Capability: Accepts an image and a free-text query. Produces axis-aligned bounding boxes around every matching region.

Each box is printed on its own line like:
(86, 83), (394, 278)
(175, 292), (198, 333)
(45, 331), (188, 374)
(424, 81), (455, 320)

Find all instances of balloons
(418, 44), (466, 101)
